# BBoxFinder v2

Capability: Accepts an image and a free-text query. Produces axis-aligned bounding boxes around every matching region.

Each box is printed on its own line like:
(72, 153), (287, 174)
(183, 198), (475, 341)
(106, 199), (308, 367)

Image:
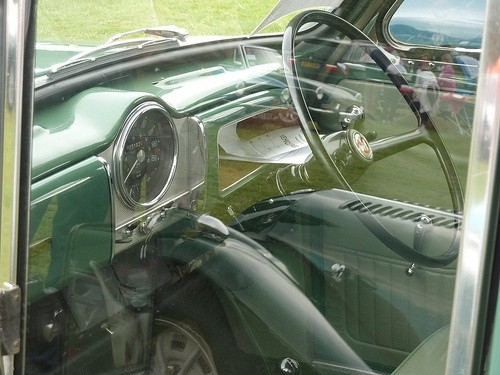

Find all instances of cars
(2, 2), (499, 375)
